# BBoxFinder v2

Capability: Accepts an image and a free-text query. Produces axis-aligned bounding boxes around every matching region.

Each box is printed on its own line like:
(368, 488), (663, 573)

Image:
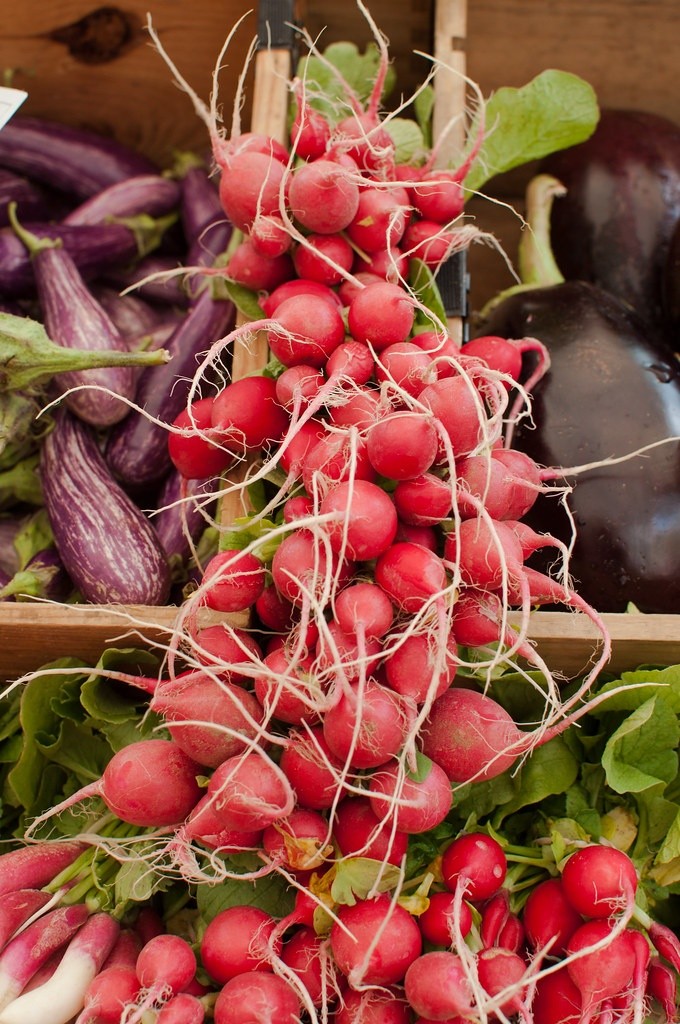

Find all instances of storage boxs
(0, 1), (680, 684)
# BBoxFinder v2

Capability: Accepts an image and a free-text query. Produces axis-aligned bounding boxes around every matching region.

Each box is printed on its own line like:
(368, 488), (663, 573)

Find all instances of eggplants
(0, 116), (240, 605)
(482, 102), (680, 615)
